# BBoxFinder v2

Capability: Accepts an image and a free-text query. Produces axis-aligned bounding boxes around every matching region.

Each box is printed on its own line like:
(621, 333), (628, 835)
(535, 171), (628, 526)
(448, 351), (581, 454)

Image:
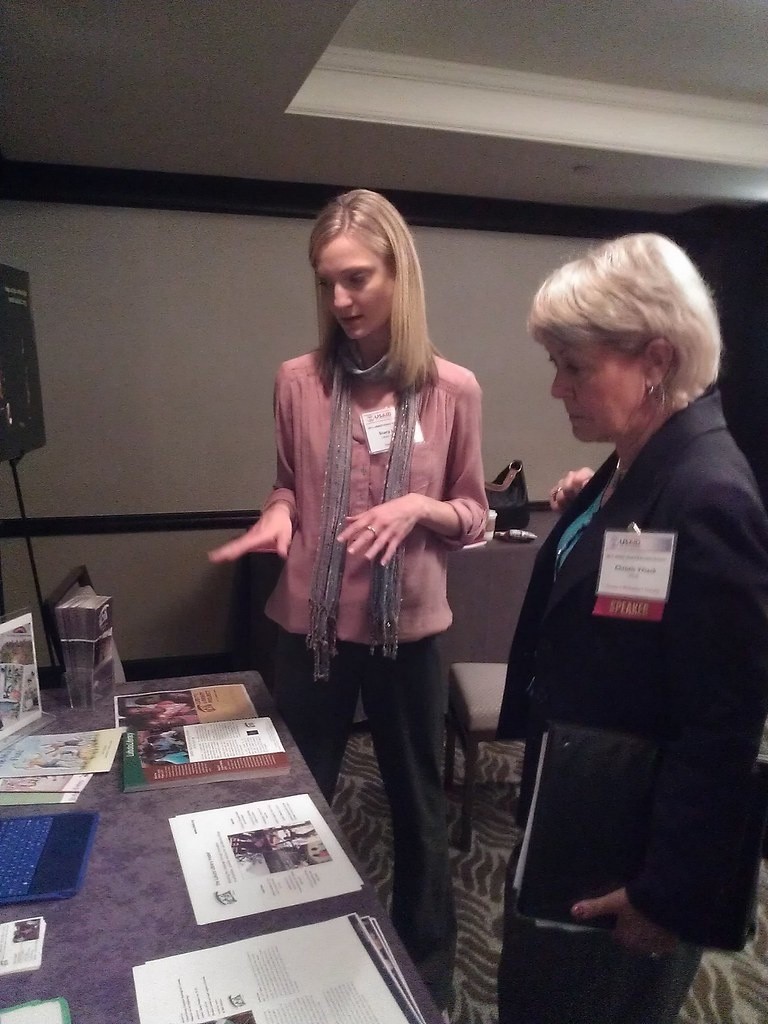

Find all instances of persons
(491, 231), (768, 1023)
(0, 666), (39, 712)
(208, 187), (490, 1024)
(121, 692), (203, 765)
(11, 737), (82, 769)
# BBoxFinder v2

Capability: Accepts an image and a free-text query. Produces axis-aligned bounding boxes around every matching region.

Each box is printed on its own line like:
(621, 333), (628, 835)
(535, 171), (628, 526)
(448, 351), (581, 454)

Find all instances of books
(0, 727), (124, 778)
(113, 684), (291, 793)
(0, 773), (94, 792)
(0, 793), (80, 806)
(56, 595), (113, 708)
(504, 724), (768, 950)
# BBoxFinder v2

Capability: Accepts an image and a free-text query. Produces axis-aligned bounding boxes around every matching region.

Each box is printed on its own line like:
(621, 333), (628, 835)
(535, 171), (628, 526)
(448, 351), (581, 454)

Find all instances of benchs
(450, 661), (510, 856)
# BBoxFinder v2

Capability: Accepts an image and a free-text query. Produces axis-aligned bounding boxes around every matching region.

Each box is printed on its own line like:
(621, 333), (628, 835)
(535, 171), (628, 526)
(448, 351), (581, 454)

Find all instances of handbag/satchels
(484, 459), (531, 532)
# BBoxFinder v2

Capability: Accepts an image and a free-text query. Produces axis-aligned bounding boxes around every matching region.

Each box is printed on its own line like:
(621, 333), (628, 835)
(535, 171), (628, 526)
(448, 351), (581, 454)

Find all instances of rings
(366, 526), (378, 538)
(553, 487), (562, 501)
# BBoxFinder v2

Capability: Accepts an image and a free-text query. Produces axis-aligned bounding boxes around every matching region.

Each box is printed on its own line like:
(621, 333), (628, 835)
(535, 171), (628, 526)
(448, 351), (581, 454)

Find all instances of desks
(0, 669), (447, 1024)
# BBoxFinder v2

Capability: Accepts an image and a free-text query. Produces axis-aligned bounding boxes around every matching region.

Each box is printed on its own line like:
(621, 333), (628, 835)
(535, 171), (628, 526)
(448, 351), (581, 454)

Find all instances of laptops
(0, 809), (100, 907)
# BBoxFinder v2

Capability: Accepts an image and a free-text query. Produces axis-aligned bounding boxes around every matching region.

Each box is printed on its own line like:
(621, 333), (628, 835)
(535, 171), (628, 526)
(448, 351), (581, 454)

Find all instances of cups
(483, 509), (497, 542)
